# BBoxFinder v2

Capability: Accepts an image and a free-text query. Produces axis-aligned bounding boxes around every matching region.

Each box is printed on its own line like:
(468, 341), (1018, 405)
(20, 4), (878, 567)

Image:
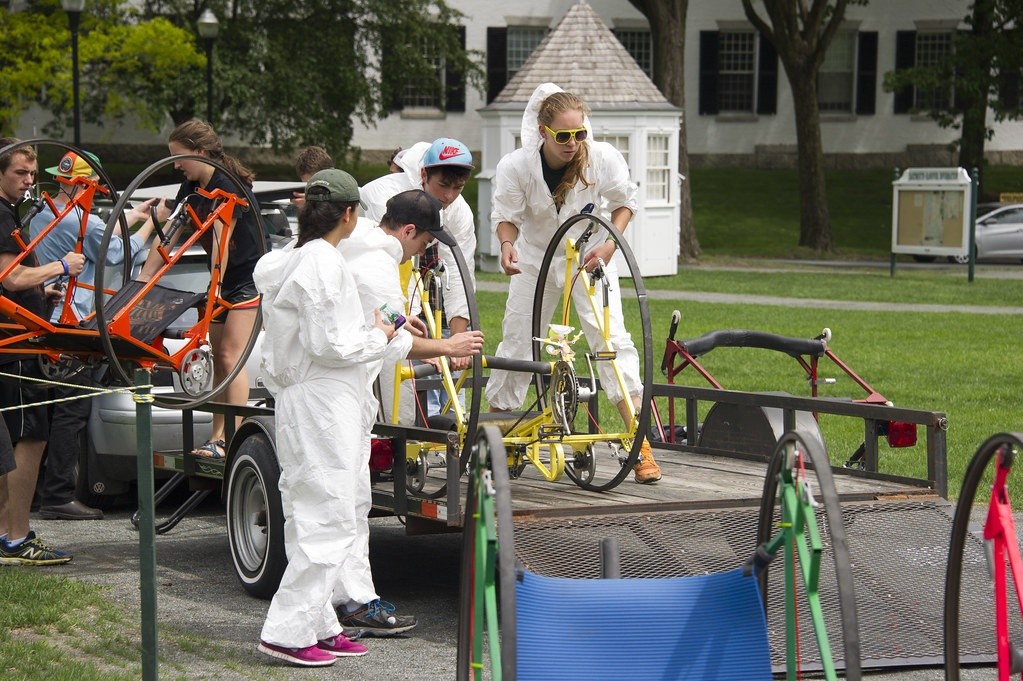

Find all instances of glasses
(545, 125), (588, 144)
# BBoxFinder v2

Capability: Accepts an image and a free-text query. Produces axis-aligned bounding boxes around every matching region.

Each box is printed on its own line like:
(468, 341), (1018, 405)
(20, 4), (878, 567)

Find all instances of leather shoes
(40, 500), (104, 521)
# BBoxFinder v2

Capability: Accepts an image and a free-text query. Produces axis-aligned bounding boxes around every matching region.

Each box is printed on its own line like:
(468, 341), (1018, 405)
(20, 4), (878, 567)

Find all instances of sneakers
(630, 441), (662, 484)
(316, 635), (368, 656)
(0, 530), (73, 565)
(257, 640), (337, 666)
(336, 600), (419, 634)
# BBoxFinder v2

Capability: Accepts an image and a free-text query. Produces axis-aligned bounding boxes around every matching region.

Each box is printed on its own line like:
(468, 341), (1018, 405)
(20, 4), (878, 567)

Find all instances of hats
(45, 150), (102, 177)
(421, 138), (476, 170)
(305, 169), (368, 211)
(387, 189), (456, 246)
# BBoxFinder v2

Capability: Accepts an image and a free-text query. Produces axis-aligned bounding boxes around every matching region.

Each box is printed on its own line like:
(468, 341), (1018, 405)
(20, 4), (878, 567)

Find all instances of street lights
(64, 0), (85, 143)
(194, 7), (222, 124)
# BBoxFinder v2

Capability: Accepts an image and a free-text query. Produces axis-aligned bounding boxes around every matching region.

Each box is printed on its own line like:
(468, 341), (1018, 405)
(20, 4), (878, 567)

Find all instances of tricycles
(453, 416), (1023, 680)
(372, 213), (654, 500)
(0, 140), (274, 412)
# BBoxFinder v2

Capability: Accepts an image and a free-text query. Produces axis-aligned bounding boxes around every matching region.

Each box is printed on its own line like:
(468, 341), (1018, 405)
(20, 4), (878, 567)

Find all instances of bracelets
(58, 259), (68, 276)
(501, 241), (513, 251)
(605, 236), (619, 249)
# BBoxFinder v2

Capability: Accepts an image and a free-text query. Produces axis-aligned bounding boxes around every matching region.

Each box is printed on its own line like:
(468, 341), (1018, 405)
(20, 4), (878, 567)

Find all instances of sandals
(188, 440), (226, 464)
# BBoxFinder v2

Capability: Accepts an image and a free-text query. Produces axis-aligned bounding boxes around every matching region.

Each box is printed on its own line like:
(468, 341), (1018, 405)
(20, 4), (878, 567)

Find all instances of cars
(908, 201), (1023, 266)
(78, 244), (265, 517)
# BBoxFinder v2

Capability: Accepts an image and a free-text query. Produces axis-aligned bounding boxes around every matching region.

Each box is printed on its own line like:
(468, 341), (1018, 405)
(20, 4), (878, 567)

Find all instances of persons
(361, 137), (477, 417)
(29, 150), (172, 520)
(485, 83), (662, 484)
(253, 168), (398, 665)
(331, 189), (485, 635)
(291, 146), (334, 208)
(135, 119), (272, 458)
(0, 137), (86, 565)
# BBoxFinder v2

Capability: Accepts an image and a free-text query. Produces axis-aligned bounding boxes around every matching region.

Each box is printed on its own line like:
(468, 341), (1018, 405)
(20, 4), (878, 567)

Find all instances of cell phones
(394, 316), (406, 331)
